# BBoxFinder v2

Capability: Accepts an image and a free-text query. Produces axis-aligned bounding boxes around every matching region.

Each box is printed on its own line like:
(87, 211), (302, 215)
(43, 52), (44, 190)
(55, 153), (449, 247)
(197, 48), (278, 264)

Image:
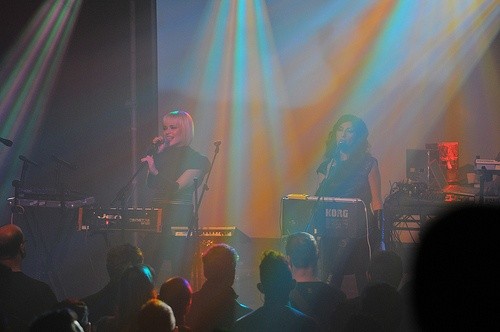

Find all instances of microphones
(334, 141), (344, 156)
(154, 140), (164, 149)
(19, 155), (38, 167)
(56, 158), (71, 166)
(0, 137), (13, 146)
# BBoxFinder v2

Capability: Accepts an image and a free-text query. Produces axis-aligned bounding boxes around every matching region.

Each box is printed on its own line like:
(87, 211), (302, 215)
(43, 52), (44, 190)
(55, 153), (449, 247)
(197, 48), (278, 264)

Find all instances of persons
(232, 250), (320, 332)
(0, 224), (59, 332)
(312, 114), (386, 298)
(138, 298), (175, 332)
(77, 243), (144, 324)
(193, 242), (255, 332)
(285, 232), (347, 332)
(138, 111), (211, 296)
(405, 200), (500, 332)
(331, 251), (415, 332)
(160, 276), (193, 314)
(94, 262), (157, 332)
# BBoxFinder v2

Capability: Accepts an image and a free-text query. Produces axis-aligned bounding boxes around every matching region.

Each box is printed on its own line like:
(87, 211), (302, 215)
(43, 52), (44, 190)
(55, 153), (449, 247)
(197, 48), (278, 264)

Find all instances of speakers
(405, 148), (438, 187)
